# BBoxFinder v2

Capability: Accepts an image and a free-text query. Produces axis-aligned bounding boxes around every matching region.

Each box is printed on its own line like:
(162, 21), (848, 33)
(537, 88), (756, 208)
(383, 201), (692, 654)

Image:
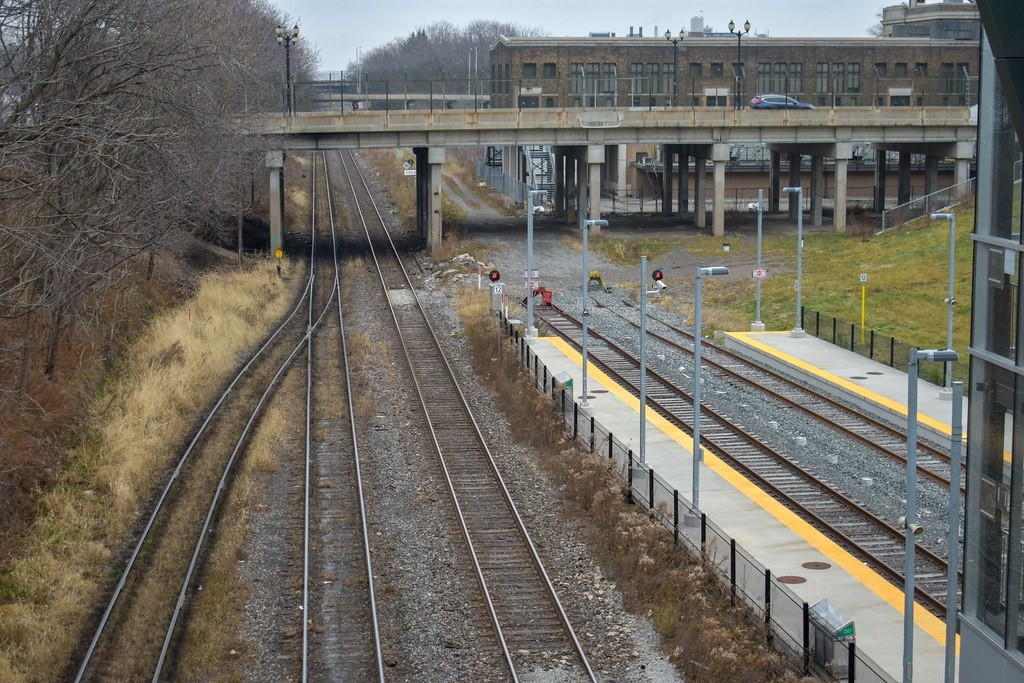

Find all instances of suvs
(752, 94), (815, 110)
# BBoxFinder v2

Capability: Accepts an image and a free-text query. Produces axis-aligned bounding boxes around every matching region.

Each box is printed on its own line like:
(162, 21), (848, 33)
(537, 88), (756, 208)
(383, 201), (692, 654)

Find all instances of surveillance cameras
(656, 280), (667, 290)
(539, 207), (544, 213)
(747, 204), (754, 211)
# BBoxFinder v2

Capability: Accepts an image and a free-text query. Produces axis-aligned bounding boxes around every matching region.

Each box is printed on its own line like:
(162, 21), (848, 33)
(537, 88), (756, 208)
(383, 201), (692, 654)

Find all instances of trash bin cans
(808, 597), (855, 680)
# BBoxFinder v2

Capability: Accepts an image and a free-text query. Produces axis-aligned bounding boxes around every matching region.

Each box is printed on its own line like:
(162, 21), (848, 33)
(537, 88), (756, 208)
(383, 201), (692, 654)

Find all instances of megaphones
(944, 299), (949, 304)
(951, 300), (957, 307)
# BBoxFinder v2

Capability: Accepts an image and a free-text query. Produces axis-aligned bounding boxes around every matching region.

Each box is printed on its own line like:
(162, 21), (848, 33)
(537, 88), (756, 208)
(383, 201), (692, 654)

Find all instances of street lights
(931, 213), (953, 392)
(527, 190), (547, 339)
(690, 266), (729, 516)
(581, 218), (608, 406)
(729, 21), (750, 110)
(665, 28), (684, 106)
(276, 23), (300, 115)
(783, 186), (802, 331)
(903, 348), (958, 683)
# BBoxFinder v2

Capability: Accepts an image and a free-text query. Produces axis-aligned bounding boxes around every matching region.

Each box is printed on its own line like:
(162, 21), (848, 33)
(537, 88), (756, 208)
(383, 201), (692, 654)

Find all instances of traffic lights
(652, 269), (664, 281)
(489, 271), (500, 281)
